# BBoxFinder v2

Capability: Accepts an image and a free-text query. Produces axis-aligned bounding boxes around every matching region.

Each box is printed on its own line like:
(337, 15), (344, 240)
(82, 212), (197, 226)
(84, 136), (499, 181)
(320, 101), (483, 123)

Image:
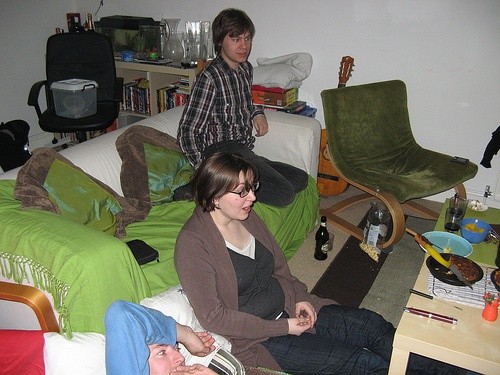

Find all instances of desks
(388, 198), (500, 375)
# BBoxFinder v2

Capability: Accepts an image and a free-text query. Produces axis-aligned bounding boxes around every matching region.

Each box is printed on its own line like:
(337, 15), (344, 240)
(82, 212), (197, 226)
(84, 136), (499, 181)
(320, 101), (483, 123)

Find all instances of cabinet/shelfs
(54, 61), (199, 143)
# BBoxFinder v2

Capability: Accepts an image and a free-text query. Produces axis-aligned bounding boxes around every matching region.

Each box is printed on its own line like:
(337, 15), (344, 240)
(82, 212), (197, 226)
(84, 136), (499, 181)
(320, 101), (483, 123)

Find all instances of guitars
(316, 55), (355, 196)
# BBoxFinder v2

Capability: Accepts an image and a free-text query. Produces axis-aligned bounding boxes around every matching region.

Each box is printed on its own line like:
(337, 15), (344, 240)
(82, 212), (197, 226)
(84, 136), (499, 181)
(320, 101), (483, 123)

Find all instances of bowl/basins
(458, 219), (491, 243)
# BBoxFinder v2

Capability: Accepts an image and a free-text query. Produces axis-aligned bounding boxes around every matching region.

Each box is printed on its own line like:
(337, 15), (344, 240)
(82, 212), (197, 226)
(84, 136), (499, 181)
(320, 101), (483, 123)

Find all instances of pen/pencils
(404, 307), (458, 325)
(409, 287), (434, 301)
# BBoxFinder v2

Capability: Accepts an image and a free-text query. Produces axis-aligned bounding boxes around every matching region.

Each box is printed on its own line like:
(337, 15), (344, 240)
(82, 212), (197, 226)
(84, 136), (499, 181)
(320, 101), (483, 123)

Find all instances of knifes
(425, 245), (473, 291)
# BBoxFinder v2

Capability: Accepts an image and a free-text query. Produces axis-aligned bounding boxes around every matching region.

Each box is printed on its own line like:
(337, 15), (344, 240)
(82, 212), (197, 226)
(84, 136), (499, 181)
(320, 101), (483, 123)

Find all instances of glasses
(227, 182), (260, 198)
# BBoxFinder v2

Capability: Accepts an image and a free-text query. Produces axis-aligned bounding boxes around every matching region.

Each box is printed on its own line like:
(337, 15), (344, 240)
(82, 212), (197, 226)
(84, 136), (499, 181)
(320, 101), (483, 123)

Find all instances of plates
(425, 252), (483, 288)
(418, 231), (473, 259)
(490, 268), (500, 293)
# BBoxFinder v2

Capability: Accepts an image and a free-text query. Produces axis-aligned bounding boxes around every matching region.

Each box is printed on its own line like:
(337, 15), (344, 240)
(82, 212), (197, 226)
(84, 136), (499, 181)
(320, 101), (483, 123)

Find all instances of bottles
(363, 187), (389, 246)
(161, 17), (214, 68)
(86, 13), (94, 32)
(314, 215), (329, 260)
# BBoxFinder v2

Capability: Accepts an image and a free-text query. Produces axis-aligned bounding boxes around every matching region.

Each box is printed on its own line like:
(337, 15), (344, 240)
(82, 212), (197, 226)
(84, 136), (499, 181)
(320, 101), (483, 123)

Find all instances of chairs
(319, 80), (478, 247)
(28, 32), (124, 144)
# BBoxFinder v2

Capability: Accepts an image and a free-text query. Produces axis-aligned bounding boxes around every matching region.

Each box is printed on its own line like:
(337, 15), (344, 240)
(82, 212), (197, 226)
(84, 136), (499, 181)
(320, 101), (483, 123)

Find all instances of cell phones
(450, 156), (469, 165)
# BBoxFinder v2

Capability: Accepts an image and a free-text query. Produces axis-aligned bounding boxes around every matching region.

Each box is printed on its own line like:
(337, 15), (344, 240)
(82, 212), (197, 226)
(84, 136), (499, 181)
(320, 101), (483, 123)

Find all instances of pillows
(116, 124), (195, 205)
(14, 147), (151, 240)
(43, 284), (232, 375)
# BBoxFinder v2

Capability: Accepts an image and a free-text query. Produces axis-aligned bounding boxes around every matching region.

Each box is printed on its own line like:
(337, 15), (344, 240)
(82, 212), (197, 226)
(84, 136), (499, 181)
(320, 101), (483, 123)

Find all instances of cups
(328, 233), (334, 251)
(444, 196), (467, 231)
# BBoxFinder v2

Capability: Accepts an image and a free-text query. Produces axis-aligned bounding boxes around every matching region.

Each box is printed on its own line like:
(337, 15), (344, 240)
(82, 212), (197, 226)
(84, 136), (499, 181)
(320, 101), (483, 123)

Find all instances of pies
(448, 256), (480, 283)
(494, 270), (500, 287)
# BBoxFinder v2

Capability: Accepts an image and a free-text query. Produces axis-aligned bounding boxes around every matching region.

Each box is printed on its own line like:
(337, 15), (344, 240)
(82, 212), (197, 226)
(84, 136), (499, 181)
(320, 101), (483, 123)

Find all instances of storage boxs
(50, 79), (98, 118)
(94, 15), (161, 58)
(251, 85), (298, 106)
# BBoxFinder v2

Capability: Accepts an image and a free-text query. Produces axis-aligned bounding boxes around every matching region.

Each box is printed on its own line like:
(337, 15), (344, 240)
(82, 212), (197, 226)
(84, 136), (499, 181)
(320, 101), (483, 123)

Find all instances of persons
(174, 153), (484, 375)
(105, 300), (219, 375)
(172, 8), (308, 206)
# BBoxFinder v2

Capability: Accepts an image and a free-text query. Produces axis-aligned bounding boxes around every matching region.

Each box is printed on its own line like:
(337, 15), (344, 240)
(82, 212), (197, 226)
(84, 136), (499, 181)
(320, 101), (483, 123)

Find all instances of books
(121, 80), (150, 113)
(156, 79), (190, 114)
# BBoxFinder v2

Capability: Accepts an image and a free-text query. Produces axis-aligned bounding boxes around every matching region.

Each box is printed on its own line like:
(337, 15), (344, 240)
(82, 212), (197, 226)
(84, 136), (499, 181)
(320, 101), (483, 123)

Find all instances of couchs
(0, 106), (321, 332)
